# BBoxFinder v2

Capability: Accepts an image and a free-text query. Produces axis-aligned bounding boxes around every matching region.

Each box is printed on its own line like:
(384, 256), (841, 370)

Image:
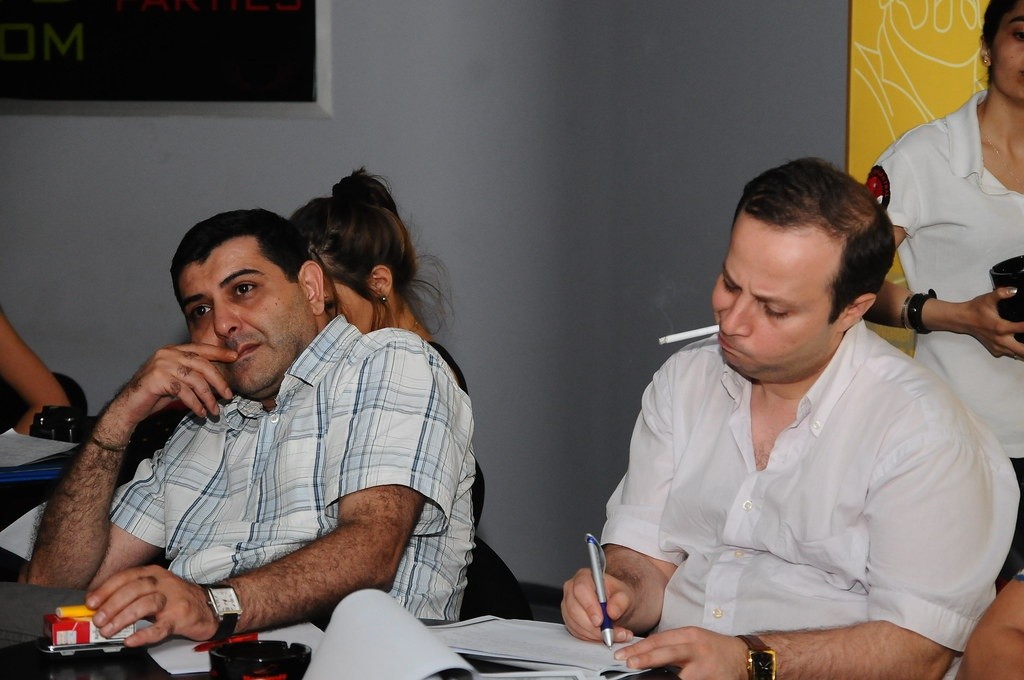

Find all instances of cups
(990, 254), (1024, 344)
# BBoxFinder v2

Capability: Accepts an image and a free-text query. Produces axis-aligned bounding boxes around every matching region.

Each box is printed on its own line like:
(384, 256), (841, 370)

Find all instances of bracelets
(88, 432), (130, 453)
(899, 288), (936, 334)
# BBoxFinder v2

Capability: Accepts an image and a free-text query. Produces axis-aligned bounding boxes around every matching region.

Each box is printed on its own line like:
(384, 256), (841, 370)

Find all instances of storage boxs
(42, 612), (136, 646)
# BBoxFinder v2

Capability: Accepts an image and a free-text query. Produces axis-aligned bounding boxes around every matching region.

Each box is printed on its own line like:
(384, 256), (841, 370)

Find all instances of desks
(0, 453), (76, 482)
(0, 582), (685, 680)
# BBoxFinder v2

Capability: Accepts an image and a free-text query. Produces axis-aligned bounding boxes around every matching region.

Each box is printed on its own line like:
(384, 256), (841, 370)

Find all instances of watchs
(736, 634), (776, 680)
(198, 583), (243, 642)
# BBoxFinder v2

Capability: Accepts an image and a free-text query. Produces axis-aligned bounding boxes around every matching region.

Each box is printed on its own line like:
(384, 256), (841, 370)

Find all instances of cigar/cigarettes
(659, 324), (719, 344)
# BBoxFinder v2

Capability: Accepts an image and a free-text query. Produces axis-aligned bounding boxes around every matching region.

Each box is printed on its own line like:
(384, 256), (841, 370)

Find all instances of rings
(1014, 354), (1018, 361)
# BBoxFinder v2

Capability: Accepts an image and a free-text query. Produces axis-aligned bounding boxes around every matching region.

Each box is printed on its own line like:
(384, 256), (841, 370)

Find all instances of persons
(20, 205), (476, 649)
(559, 156), (996, 680)
(956, 563), (1024, 680)
(866, 0), (1024, 590)
(284, 165), (485, 534)
(0, 308), (71, 436)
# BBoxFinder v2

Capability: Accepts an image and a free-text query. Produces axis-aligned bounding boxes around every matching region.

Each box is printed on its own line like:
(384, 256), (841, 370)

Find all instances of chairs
(0, 370), (86, 433)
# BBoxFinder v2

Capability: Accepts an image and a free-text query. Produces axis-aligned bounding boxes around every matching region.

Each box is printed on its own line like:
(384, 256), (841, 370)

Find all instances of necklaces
(409, 320), (419, 331)
(978, 121), (1024, 192)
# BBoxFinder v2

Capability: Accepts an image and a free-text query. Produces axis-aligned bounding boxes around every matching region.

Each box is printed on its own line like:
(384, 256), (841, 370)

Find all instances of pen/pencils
(584, 532), (614, 651)
(189, 631), (259, 652)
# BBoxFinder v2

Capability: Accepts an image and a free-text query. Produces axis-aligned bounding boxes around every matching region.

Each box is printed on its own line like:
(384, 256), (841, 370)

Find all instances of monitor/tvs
(0, 0), (333, 118)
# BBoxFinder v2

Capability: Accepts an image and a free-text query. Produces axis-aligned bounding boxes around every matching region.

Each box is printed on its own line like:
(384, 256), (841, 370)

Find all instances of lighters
(55, 605), (97, 618)
